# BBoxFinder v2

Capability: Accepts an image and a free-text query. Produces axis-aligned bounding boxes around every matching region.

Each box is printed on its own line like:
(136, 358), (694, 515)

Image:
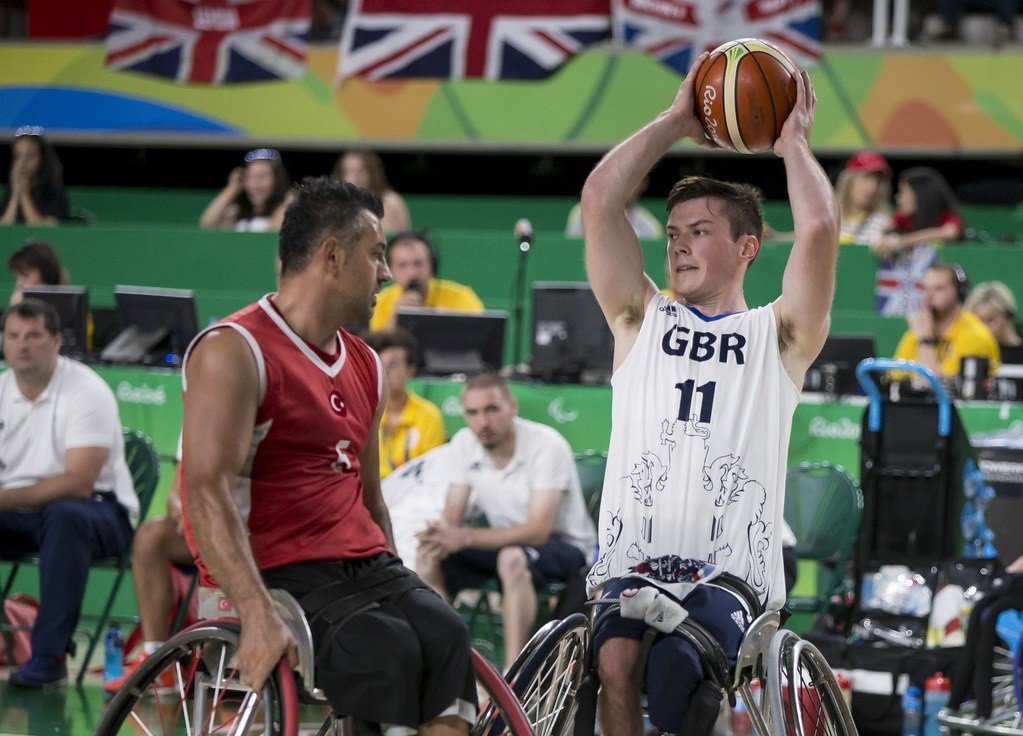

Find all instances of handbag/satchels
(0, 595), (40, 665)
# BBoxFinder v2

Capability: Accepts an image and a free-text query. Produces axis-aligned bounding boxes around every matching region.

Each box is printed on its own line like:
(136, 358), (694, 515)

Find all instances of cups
(959, 356), (988, 400)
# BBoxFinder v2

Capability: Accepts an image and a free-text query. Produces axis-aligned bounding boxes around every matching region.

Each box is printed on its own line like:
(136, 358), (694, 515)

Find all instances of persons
(885, 262), (1000, 401)
(104, 423), (195, 698)
(0, 299), (141, 688)
(361, 328), (445, 479)
(200, 148), (294, 233)
(767, 151), (893, 247)
(565, 174), (663, 240)
(333, 147), (412, 231)
(179, 174), (480, 736)
(0, 125), (70, 226)
(869, 165), (963, 258)
(964, 280), (1023, 401)
(368, 232), (486, 331)
(414, 374), (597, 678)
(0, 244), (94, 350)
(580, 51), (841, 736)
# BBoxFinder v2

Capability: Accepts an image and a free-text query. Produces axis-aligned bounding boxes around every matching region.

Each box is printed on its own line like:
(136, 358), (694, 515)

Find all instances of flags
(616, 0), (822, 79)
(102, 0), (312, 87)
(339, 0), (611, 81)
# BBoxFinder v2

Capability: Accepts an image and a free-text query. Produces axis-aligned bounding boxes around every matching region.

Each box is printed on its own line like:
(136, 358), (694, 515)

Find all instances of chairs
(0, 427), (161, 685)
(780, 462), (864, 627)
(466, 450), (607, 646)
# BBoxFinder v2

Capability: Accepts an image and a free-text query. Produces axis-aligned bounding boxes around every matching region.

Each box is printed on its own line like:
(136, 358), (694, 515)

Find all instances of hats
(847, 153), (891, 177)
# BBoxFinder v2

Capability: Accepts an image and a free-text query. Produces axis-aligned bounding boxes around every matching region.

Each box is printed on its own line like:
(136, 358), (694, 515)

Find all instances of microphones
(514, 218), (532, 242)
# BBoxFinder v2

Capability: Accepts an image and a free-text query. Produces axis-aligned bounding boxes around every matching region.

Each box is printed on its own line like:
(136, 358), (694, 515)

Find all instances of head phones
(949, 267), (970, 300)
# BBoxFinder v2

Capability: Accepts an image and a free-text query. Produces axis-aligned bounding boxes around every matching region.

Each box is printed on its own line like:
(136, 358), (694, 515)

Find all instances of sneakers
(101, 645), (186, 695)
(8, 654), (71, 690)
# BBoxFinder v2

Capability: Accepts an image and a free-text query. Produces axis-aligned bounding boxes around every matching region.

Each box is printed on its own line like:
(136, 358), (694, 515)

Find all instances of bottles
(102, 621), (125, 701)
(924, 670), (952, 735)
(903, 686), (924, 735)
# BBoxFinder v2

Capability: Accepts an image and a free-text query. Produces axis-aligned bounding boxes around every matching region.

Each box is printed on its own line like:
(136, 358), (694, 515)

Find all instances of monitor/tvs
(528, 282), (614, 384)
(99, 284), (201, 369)
(804, 336), (876, 394)
(22, 286), (90, 360)
(396, 309), (507, 380)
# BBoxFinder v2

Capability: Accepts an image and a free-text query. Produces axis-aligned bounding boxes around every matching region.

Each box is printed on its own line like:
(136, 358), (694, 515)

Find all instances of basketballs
(695, 38), (801, 155)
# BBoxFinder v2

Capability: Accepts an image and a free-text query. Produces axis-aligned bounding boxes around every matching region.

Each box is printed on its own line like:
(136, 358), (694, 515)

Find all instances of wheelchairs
(94, 564), (540, 736)
(784, 361), (1023, 736)
(470, 567), (861, 736)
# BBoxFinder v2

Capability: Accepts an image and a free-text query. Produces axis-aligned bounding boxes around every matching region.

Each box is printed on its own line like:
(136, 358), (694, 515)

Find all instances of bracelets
(921, 338), (936, 344)
